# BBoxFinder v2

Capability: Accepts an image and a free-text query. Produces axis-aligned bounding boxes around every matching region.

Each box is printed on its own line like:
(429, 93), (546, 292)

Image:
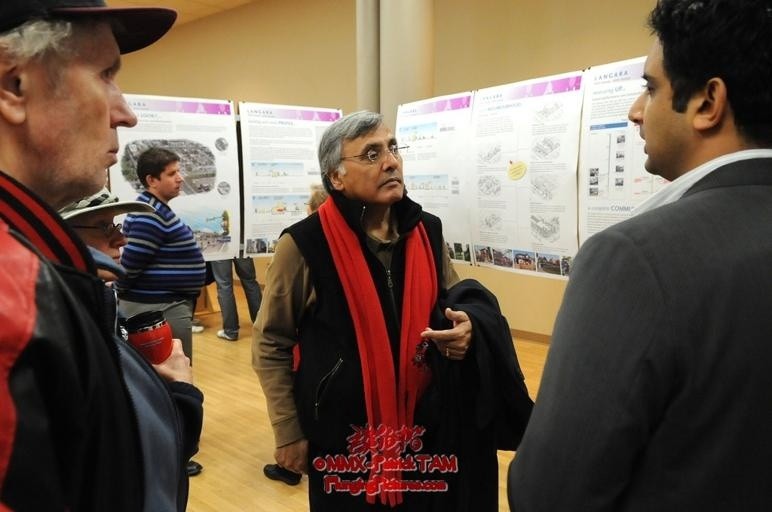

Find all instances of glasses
(340, 143), (411, 163)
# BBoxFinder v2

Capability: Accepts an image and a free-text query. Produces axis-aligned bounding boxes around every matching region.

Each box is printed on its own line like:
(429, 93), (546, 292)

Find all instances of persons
(107, 142), (204, 480)
(0, 0), (184, 512)
(247, 108), (500, 512)
(505, 0), (771, 511)
(191, 261), (207, 336)
(262, 181), (330, 487)
(52, 182), (206, 512)
(210, 258), (264, 343)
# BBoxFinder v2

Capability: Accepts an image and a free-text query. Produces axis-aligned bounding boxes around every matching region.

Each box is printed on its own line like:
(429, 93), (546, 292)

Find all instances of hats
(1, 0), (180, 56)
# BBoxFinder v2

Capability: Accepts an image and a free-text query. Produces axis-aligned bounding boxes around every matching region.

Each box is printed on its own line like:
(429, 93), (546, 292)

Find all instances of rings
(439, 344), (450, 357)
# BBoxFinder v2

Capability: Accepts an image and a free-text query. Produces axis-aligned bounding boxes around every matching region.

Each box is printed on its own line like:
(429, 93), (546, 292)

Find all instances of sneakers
(216, 329), (238, 341)
(186, 460), (202, 476)
(264, 463), (303, 485)
(192, 325), (204, 333)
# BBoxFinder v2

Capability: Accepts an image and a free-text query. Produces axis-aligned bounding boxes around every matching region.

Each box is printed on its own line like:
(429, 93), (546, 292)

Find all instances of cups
(121, 308), (176, 367)
